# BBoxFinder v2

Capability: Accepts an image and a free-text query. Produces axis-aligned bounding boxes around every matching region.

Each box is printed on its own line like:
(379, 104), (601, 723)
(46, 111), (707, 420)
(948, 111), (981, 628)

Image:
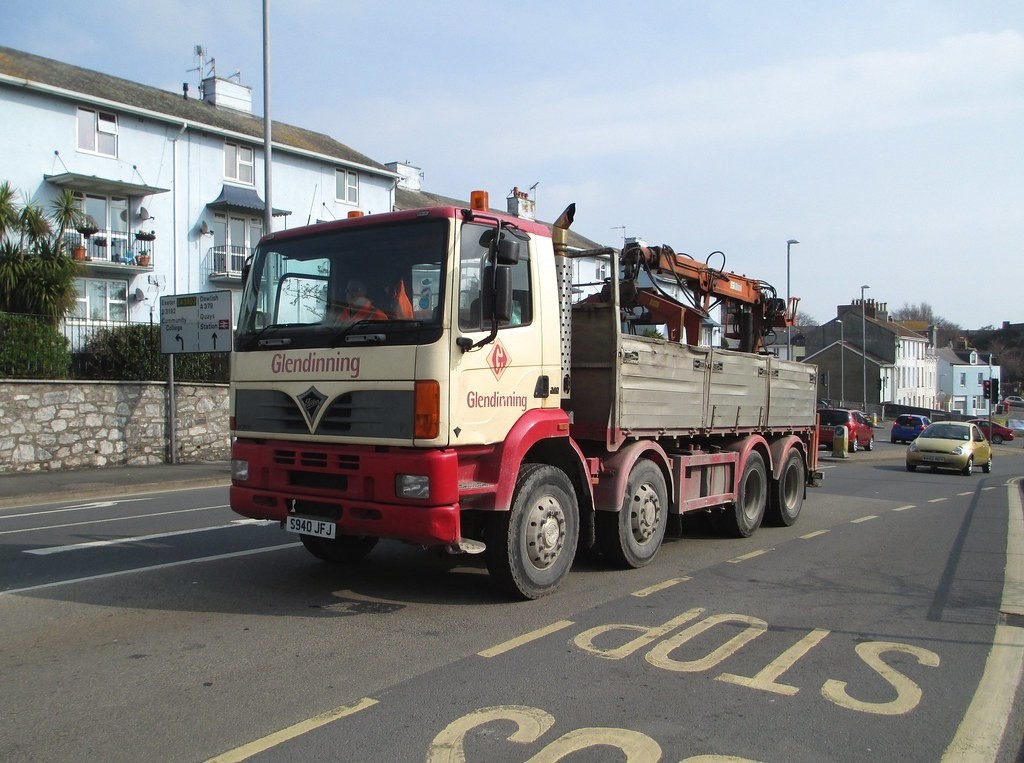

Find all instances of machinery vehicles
(576, 241), (803, 356)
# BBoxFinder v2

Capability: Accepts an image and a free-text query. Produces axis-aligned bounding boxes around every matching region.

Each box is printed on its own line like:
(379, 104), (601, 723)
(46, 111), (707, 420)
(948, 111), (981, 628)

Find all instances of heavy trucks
(229, 186), (825, 601)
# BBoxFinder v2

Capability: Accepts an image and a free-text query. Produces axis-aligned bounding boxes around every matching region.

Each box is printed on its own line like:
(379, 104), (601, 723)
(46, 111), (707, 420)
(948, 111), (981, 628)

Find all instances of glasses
(345, 286), (366, 292)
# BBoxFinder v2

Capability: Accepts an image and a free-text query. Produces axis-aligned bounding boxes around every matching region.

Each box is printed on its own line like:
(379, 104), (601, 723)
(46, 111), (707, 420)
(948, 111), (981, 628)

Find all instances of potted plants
(78, 224), (99, 240)
(139, 249), (151, 266)
(136, 231), (156, 241)
(73, 242), (86, 261)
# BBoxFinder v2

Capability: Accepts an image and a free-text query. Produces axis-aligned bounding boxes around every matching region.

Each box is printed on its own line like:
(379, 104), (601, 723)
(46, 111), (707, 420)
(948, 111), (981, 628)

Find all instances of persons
(335, 279), (388, 324)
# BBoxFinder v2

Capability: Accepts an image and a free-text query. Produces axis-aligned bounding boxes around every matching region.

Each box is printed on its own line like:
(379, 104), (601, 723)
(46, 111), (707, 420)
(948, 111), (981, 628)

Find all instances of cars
(966, 419), (1015, 445)
(858, 409), (873, 428)
(1004, 395), (1024, 407)
(890, 413), (932, 445)
(906, 421), (992, 476)
(815, 407), (874, 451)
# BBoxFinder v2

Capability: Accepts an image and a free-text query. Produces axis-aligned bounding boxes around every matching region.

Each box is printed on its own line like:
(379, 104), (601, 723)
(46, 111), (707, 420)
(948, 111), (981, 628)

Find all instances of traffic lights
(983, 380), (992, 399)
(992, 378), (1001, 404)
(820, 373), (826, 386)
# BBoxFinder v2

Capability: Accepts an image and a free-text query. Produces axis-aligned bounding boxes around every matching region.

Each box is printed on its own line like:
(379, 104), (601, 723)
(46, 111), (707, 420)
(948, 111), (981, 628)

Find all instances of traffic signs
(160, 290), (232, 354)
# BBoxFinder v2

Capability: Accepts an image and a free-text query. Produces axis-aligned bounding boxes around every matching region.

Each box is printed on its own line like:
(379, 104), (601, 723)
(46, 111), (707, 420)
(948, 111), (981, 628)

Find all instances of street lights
(859, 285), (871, 413)
(784, 239), (801, 361)
